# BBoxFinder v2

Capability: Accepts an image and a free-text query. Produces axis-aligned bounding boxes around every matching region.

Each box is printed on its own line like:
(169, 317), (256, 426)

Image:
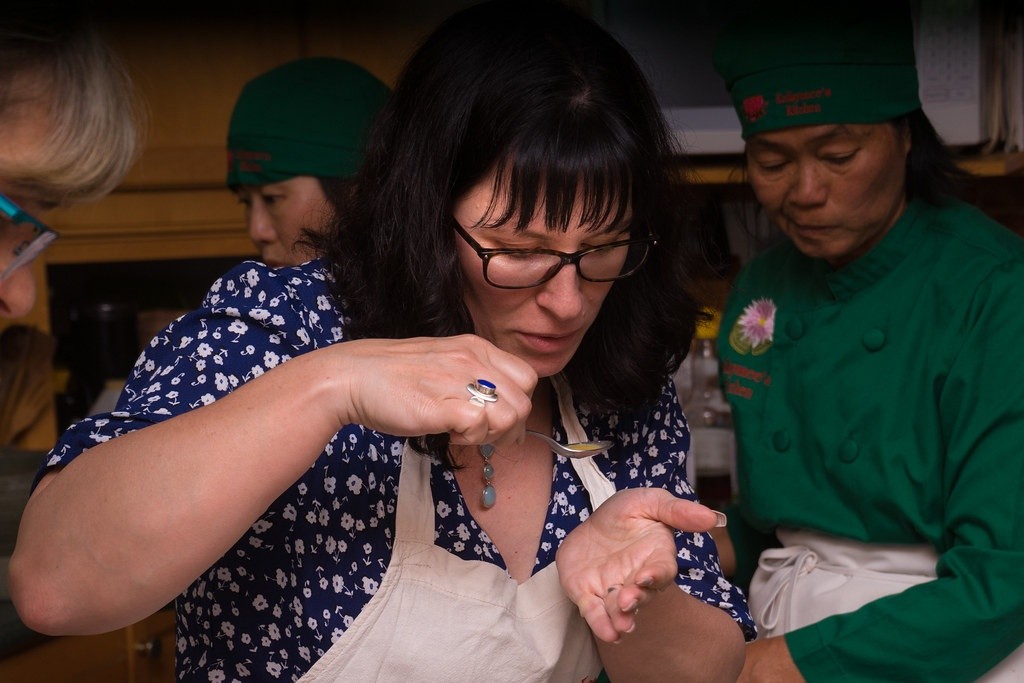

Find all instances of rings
(604, 584), (639, 617)
(467, 378), (498, 408)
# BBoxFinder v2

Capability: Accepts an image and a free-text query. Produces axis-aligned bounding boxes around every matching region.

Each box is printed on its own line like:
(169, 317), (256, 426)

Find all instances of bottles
(678, 334), (738, 479)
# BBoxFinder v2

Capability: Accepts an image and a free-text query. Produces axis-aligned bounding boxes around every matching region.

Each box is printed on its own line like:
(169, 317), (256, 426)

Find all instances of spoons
(521, 430), (615, 458)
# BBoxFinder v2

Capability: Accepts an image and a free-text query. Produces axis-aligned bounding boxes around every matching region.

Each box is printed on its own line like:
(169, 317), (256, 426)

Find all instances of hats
(225, 54), (400, 188)
(711, 1), (923, 137)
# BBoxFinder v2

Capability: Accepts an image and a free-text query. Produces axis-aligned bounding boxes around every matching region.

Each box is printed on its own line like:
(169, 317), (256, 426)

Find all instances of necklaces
(479, 442), (496, 508)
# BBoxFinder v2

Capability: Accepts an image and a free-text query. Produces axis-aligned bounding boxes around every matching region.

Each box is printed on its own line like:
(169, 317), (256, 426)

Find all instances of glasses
(447, 211), (657, 290)
(0, 194), (61, 280)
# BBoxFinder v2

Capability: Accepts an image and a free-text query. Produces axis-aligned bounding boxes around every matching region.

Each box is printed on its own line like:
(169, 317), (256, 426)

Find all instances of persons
(9, 0), (759, 683)
(0, 29), (150, 316)
(226, 57), (392, 269)
(706, 0), (1024, 683)
